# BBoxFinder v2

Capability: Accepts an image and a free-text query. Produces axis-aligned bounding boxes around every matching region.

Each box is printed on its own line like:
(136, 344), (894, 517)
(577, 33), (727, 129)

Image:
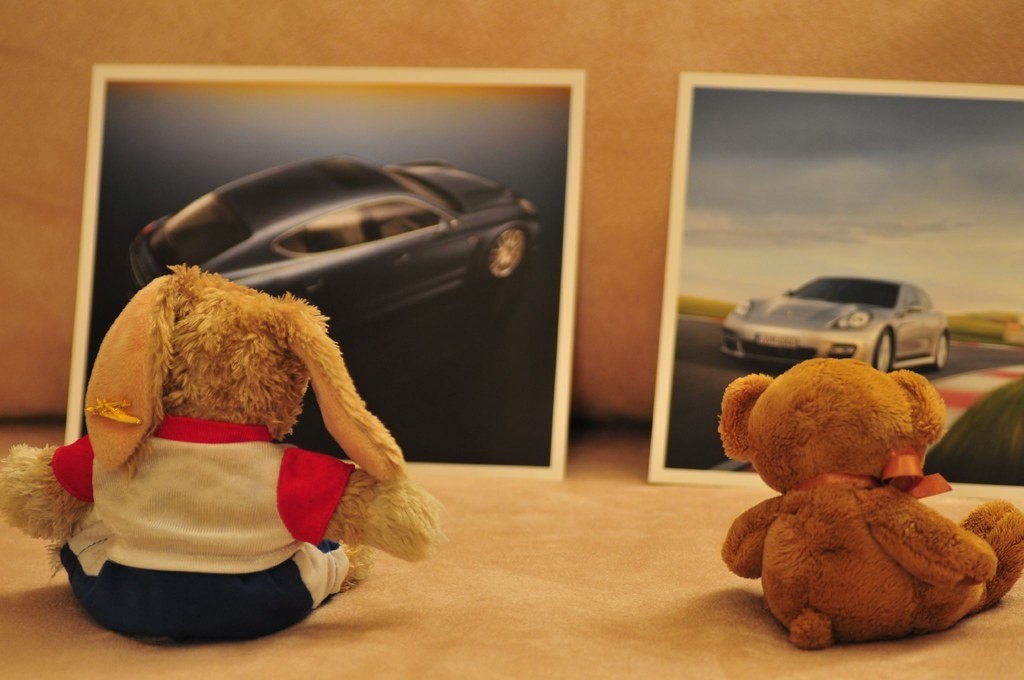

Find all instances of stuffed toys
(0, 264), (448, 648)
(718, 358), (1024, 653)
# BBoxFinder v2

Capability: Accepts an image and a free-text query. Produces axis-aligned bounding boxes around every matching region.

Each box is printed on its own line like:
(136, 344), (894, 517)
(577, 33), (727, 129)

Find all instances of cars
(718, 275), (951, 372)
(128, 154), (543, 333)
